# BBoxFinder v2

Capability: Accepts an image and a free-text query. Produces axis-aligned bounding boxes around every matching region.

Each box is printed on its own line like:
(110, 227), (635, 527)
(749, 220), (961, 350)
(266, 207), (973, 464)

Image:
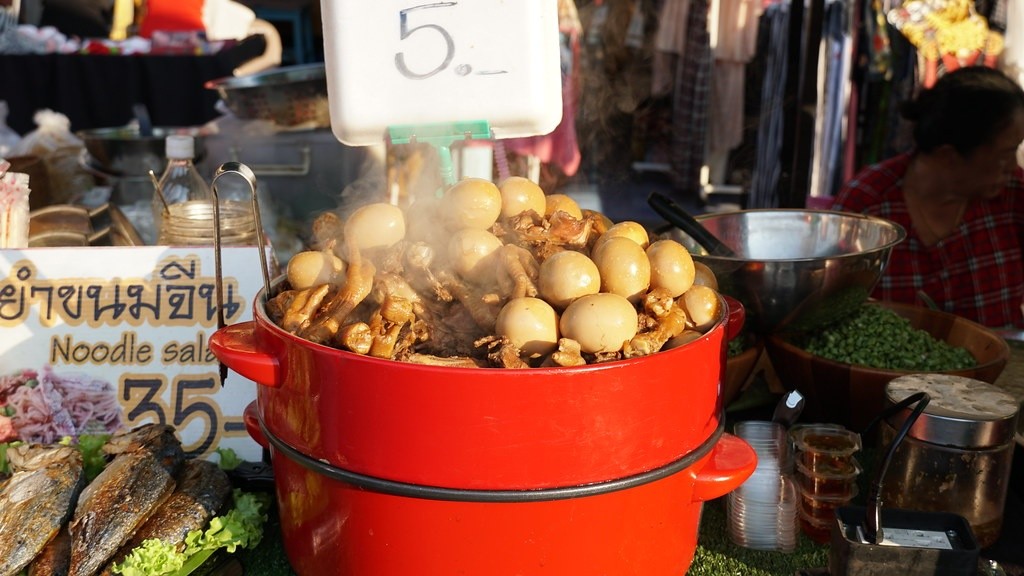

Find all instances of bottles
(152, 136), (212, 246)
(157, 199), (282, 279)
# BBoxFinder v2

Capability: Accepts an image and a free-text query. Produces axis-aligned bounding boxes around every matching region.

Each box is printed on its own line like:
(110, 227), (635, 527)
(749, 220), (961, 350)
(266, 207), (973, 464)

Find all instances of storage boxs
(200, 108), (348, 220)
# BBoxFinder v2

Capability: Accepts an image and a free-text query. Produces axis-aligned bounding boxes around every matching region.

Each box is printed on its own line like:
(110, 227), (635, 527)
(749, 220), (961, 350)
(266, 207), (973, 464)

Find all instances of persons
(832, 66), (1024, 328)
(40, 0), (282, 77)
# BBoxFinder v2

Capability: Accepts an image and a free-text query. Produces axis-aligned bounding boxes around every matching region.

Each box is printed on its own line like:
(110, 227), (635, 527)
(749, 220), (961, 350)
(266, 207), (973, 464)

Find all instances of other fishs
(0, 423), (232, 576)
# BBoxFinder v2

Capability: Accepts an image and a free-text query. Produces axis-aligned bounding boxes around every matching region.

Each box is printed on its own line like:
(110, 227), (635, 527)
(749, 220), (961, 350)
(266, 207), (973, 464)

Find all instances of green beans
(797, 299), (980, 373)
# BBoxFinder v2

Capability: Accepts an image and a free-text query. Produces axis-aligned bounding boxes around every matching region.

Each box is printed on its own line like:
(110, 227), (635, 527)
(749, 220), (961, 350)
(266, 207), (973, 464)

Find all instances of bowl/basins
(654, 208), (906, 330)
(766, 303), (1012, 425)
(79, 129), (203, 205)
(725, 331), (765, 405)
(204, 65), (330, 131)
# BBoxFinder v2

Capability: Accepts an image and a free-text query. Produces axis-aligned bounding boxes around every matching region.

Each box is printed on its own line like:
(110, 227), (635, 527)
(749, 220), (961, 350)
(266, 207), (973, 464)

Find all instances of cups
(0, 172), (32, 249)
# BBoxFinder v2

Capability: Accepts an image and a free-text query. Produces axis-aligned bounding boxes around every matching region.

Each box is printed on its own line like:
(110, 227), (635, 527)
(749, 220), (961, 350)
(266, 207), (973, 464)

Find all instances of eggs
(285, 174), (723, 357)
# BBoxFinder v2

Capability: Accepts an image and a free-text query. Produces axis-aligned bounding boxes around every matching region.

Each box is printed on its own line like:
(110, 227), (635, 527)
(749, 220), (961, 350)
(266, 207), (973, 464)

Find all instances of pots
(209, 271), (758, 576)
(0, 32), (268, 135)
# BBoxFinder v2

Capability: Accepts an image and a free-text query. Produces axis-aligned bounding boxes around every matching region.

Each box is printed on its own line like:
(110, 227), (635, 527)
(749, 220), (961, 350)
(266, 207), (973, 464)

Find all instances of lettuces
(0, 434), (270, 576)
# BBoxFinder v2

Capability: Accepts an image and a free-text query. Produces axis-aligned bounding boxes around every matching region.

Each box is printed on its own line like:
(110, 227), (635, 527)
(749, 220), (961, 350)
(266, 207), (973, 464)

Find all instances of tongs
(213, 163), (271, 386)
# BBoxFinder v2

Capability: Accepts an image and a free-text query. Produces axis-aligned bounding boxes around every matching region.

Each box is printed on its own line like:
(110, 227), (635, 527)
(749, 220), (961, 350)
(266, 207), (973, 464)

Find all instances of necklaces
(915, 194), (963, 239)
